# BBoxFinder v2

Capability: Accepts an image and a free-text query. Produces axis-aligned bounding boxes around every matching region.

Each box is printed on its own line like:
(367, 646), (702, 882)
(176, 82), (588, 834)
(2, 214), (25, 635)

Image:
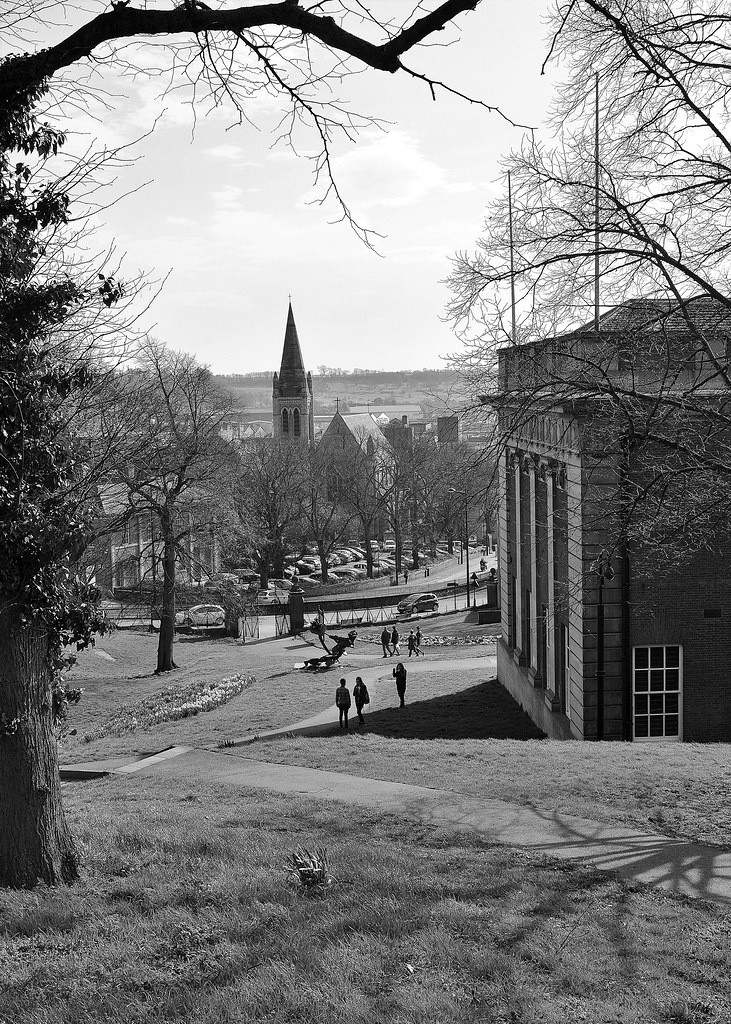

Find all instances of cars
(397, 593), (438, 613)
(292, 546), (446, 589)
(282, 545), (366, 577)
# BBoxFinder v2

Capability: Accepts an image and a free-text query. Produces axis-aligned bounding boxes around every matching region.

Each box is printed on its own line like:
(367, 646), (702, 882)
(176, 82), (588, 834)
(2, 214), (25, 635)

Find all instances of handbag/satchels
(396, 644), (400, 650)
(362, 684), (369, 704)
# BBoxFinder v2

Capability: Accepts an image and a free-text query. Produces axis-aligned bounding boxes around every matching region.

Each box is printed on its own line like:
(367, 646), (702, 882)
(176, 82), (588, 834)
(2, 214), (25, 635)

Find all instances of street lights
(448, 488), (471, 608)
(596, 550), (615, 740)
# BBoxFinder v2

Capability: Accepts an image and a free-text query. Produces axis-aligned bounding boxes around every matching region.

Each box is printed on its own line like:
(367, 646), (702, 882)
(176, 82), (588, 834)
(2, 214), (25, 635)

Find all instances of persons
(405, 625), (425, 657)
(393, 663), (407, 708)
(480, 558), (487, 570)
(336, 678), (351, 728)
(381, 627), (393, 658)
(391, 626), (400, 656)
(348, 630), (357, 649)
(318, 605), (324, 624)
(404, 566), (409, 584)
(353, 677), (367, 725)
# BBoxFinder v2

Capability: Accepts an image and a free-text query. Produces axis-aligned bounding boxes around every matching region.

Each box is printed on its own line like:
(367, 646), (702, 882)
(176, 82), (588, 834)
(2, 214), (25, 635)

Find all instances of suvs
(174, 604), (225, 627)
(257, 590), (289, 604)
